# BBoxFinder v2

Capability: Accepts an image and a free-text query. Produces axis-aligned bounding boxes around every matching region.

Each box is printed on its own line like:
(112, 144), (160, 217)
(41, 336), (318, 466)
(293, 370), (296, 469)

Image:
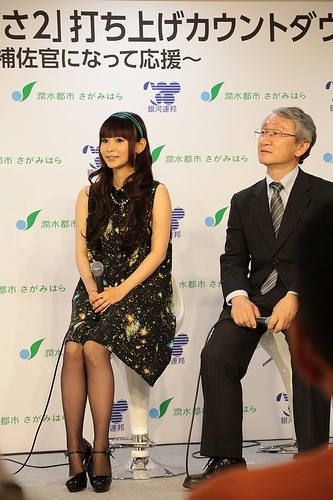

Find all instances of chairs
(256, 330), (295, 454)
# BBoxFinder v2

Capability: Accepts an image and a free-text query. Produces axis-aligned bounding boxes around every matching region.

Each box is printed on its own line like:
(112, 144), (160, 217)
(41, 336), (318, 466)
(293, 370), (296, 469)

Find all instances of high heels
(65, 439), (113, 493)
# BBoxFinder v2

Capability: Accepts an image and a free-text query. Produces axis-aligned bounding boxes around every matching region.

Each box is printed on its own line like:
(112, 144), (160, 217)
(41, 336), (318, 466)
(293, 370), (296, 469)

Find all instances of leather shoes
(183, 457), (247, 488)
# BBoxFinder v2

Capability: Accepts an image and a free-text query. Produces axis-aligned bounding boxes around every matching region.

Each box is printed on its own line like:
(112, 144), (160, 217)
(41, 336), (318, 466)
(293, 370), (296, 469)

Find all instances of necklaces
(111, 193), (131, 205)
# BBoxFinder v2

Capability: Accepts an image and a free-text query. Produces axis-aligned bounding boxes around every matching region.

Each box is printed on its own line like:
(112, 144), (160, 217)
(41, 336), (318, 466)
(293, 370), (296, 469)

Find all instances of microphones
(229, 316), (272, 326)
(91, 261), (104, 294)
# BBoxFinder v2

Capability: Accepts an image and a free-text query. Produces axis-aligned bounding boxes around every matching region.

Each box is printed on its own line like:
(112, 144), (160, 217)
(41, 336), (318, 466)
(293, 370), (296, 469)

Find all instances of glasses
(253, 128), (299, 141)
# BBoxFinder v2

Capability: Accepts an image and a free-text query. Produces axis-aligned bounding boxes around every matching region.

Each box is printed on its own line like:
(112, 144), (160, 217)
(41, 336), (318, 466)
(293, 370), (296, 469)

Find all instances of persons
(186, 201), (333, 500)
(182, 107), (333, 489)
(61, 112), (177, 493)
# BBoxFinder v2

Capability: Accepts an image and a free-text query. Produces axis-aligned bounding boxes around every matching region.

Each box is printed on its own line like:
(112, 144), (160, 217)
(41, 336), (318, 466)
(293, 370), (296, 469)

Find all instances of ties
(261, 183), (284, 295)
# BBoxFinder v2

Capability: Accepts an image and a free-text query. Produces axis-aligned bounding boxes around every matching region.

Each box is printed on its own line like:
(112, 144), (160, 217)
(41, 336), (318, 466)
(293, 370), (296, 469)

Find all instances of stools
(113, 278), (185, 479)
